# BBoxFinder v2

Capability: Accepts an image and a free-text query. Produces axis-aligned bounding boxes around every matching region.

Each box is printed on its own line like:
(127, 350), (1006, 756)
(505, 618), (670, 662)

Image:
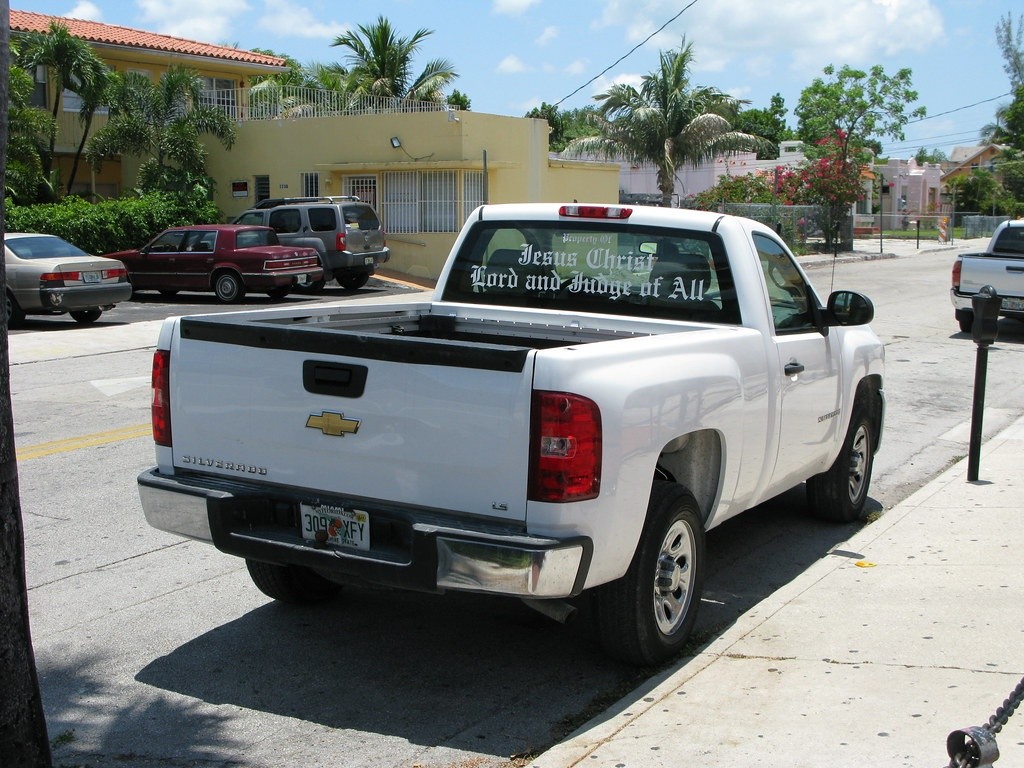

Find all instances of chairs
(491, 246), (725, 324)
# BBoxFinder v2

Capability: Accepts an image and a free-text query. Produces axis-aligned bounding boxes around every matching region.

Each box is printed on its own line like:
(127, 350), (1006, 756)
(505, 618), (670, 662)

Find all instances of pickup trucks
(135, 196), (893, 663)
(949, 221), (1024, 336)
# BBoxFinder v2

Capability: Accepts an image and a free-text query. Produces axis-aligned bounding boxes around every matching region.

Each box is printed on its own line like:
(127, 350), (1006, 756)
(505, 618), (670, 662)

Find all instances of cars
(5, 232), (133, 328)
(101, 223), (323, 303)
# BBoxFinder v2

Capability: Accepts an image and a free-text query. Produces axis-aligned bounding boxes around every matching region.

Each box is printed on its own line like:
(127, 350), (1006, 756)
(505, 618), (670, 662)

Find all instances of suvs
(226, 196), (391, 291)
